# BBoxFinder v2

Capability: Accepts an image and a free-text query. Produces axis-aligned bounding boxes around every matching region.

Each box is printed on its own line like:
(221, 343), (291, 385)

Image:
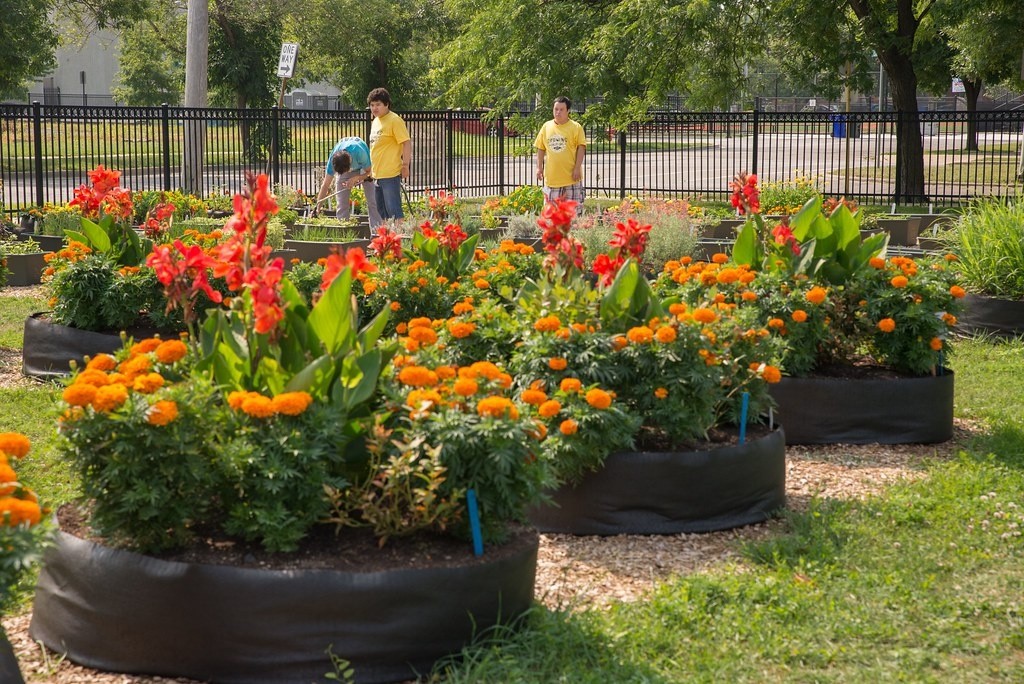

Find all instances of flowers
(0, 163), (968, 649)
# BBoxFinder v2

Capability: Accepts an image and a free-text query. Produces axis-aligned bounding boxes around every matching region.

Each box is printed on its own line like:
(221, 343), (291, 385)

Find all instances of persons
(533, 96), (586, 218)
(367, 88), (411, 221)
(316, 136), (385, 240)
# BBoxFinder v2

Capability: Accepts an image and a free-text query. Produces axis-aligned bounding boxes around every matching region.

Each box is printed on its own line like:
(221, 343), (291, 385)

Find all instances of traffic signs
(277, 41), (299, 77)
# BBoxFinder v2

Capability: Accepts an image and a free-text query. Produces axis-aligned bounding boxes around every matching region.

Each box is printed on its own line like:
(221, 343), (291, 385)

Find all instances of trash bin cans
(829, 115), (847, 138)
(848, 114), (864, 139)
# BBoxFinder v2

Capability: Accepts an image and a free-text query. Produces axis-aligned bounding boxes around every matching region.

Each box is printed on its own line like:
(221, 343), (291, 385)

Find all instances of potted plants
(859, 216), (884, 243)
(918, 176), (1024, 346)
(869, 211), (923, 247)
(284, 225), (370, 267)
(287, 211), (371, 238)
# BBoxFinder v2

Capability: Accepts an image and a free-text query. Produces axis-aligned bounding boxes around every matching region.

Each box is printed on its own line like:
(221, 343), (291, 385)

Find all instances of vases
(514, 237), (546, 256)
(283, 229), (291, 239)
(26, 497), (539, 684)
(479, 227), (508, 244)
(736, 215), (785, 221)
(350, 214), (369, 222)
(470, 216), (508, 227)
(207, 209), (234, 219)
(917, 237), (951, 251)
(173, 222), (224, 232)
(765, 356), (953, 448)
(519, 410), (786, 539)
(328, 216), (361, 222)
(371, 233), (414, 253)
(696, 220), (764, 241)
(886, 214), (953, 237)
(20, 231), (68, 253)
(6, 251), (52, 286)
(22, 311), (200, 393)
(293, 208), (336, 217)
(269, 249), (298, 272)
(694, 242), (734, 262)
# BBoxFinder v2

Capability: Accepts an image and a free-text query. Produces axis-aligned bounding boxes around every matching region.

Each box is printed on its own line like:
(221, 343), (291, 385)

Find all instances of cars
(444, 104), (521, 138)
